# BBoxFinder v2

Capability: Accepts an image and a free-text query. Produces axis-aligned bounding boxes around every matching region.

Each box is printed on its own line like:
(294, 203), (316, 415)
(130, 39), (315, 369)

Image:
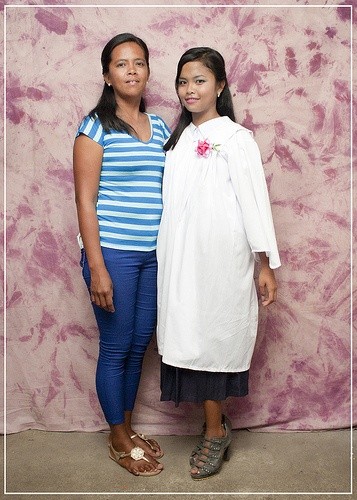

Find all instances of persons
(72, 32), (171, 477)
(154, 47), (284, 481)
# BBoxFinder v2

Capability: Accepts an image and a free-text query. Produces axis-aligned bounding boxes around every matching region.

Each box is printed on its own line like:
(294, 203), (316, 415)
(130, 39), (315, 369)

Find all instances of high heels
(190, 423), (232, 481)
(189, 414), (232, 464)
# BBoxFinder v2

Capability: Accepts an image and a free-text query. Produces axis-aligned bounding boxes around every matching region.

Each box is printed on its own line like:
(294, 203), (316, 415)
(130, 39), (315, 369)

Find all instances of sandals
(130, 432), (164, 459)
(108, 441), (163, 476)
